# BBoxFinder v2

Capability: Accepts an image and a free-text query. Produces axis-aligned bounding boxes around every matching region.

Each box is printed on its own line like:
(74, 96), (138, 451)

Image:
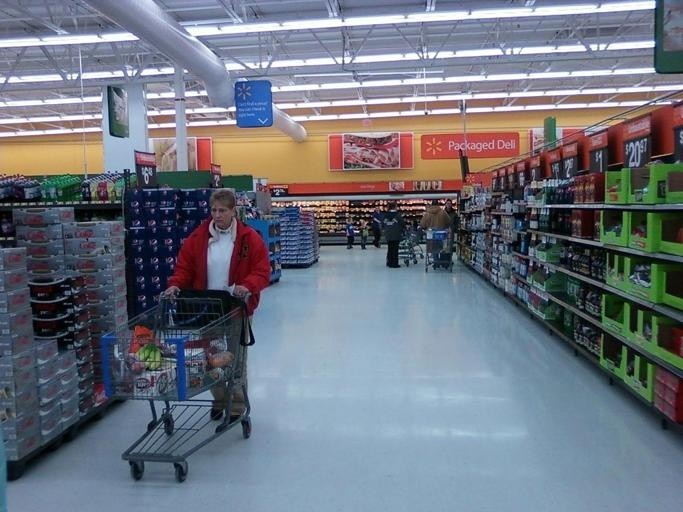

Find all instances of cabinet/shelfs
(245, 218), (282, 285)
(454, 86), (682, 429)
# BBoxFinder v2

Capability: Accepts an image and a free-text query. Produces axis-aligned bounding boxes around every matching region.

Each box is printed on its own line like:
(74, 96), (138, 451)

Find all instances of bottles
(525, 172), (604, 360)
(0, 172), (137, 203)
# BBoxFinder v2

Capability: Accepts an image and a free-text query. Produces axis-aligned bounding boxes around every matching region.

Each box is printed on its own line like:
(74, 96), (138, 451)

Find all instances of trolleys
(98, 290), (252, 482)
(397, 225), (455, 273)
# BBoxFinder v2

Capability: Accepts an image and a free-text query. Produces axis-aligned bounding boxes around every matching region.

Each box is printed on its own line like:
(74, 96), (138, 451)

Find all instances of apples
(127, 352), (143, 375)
(122, 375), (133, 392)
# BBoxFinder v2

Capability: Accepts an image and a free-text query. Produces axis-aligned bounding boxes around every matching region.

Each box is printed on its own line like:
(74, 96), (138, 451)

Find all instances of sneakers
(210, 408), (243, 425)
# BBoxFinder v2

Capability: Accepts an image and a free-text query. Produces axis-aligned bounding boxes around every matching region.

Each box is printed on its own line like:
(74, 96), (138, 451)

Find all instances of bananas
(136, 340), (162, 370)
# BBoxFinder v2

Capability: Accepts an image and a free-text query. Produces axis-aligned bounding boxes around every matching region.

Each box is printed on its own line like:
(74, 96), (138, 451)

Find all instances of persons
(444, 198), (460, 265)
(360, 219), (368, 250)
(372, 206), (384, 248)
(163, 188), (272, 425)
(345, 217), (355, 249)
(382, 203), (404, 268)
(419, 200), (452, 268)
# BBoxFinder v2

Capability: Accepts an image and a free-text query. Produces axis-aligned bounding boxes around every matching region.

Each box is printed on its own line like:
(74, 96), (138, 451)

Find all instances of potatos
(209, 350), (235, 368)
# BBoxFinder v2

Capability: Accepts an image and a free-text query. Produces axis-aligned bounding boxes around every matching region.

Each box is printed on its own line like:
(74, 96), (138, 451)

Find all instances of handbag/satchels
(174, 288), (231, 327)
